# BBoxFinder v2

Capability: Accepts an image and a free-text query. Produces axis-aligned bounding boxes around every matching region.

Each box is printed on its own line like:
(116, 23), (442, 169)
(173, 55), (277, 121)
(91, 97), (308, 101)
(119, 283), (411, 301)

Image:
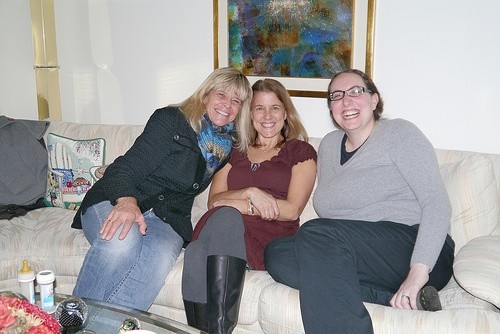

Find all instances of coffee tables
(34, 292), (210, 334)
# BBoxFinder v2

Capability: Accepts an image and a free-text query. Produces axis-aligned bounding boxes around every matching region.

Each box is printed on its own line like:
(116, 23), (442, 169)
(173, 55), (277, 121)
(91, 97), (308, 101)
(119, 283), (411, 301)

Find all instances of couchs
(0, 116), (500, 334)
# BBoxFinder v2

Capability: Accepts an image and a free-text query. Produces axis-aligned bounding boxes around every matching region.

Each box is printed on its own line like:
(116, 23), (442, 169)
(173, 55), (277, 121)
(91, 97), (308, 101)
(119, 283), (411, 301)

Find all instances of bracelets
(247, 197), (254, 216)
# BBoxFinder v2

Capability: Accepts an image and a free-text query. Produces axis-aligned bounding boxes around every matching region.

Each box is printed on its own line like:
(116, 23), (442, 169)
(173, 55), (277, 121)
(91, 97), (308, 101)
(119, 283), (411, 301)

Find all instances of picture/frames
(213, 0), (376, 97)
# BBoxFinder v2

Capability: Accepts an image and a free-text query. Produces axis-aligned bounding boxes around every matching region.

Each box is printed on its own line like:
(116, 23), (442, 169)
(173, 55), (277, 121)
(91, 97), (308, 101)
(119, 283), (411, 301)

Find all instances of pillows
(44, 133), (107, 211)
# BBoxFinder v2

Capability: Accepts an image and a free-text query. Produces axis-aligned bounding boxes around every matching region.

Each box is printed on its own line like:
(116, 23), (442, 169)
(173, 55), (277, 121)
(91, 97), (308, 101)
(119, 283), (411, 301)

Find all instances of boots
(206, 255), (247, 334)
(184, 299), (209, 331)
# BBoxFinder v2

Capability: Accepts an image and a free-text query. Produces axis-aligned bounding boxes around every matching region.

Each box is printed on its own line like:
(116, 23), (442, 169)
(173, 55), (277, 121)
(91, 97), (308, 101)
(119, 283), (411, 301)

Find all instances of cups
(37, 270), (56, 310)
(122, 330), (157, 334)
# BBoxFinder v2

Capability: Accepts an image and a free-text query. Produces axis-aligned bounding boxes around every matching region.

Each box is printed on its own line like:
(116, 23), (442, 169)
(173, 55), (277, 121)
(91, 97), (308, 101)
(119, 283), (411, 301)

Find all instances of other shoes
(416, 285), (442, 312)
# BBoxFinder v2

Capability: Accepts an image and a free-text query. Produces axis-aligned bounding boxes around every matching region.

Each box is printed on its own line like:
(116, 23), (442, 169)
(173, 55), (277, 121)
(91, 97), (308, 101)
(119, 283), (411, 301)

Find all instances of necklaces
(252, 137), (286, 153)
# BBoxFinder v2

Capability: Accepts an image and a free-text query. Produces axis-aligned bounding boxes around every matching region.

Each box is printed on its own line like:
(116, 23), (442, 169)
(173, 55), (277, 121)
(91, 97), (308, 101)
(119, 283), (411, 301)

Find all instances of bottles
(17, 260), (35, 305)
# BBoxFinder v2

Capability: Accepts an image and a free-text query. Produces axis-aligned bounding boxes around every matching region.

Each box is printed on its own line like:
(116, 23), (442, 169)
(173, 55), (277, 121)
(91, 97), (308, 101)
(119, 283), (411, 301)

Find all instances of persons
(263, 69), (455, 334)
(70, 68), (253, 334)
(181, 79), (317, 334)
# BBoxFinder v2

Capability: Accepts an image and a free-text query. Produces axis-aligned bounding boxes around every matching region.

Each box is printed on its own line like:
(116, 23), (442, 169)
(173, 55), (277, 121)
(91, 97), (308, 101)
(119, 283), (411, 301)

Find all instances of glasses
(328, 86), (373, 101)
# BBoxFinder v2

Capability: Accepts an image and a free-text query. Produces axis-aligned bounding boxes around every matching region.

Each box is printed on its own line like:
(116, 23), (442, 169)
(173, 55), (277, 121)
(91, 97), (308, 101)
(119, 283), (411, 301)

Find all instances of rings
(106, 217), (110, 221)
(109, 220), (113, 223)
(402, 295), (409, 299)
